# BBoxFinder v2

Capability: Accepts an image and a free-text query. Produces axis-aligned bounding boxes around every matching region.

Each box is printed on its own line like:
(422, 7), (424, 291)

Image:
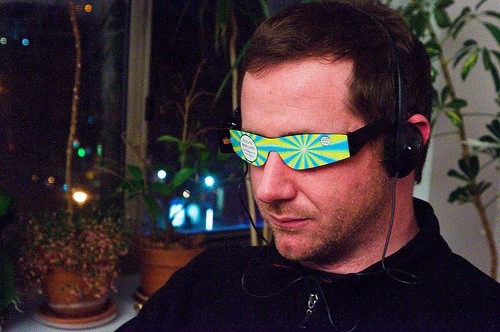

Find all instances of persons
(113, 0), (500, 332)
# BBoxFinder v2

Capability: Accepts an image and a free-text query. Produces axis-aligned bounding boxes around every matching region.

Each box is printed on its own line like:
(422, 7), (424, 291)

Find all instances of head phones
(355, 7), (424, 179)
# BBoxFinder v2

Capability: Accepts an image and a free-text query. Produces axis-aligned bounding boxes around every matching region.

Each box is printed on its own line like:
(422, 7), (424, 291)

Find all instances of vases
(40, 269), (117, 324)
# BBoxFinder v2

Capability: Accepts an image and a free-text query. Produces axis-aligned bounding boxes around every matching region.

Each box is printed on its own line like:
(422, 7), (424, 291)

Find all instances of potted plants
(107, 121), (231, 303)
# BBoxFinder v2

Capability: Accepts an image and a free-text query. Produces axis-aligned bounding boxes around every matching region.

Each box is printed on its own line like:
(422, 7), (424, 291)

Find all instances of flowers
(11, 207), (131, 298)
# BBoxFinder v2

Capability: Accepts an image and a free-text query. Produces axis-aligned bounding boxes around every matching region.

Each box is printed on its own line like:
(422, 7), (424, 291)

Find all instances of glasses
(229, 112), (407, 171)
